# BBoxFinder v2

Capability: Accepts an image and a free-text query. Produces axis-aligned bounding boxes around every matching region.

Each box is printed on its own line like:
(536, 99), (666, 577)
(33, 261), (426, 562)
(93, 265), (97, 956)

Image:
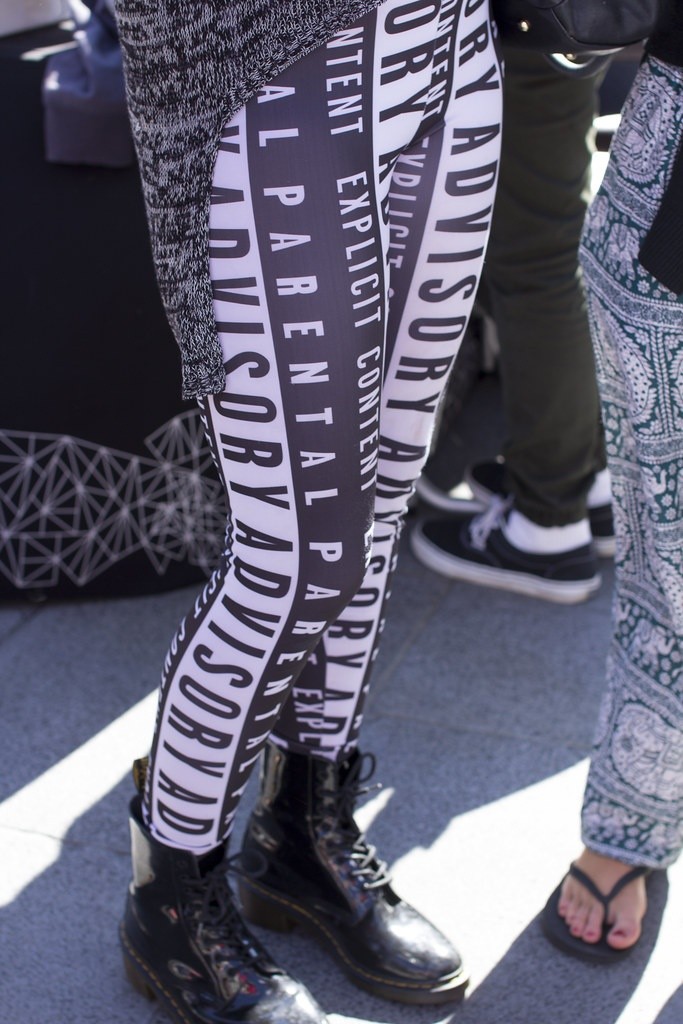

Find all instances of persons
(412, 1), (611, 604)
(541, 0), (683, 968)
(114, 1), (505, 1024)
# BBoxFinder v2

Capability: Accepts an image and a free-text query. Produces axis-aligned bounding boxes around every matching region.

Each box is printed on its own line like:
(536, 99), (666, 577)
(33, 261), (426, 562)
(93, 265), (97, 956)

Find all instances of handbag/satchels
(489, 0), (660, 54)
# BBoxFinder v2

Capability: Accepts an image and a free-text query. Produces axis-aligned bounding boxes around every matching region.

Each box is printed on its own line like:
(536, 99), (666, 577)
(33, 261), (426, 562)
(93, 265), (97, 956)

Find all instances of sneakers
(465, 459), (617, 559)
(410, 512), (603, 603)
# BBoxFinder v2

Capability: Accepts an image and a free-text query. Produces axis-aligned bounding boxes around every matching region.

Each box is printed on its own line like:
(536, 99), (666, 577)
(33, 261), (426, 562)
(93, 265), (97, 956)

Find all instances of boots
(116, 791), (328, 1023)
(227, 741), (471, 1007)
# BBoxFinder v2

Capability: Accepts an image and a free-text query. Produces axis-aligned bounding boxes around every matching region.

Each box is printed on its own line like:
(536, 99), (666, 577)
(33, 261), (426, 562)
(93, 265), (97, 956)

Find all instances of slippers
(542, 858), (656, 965)
